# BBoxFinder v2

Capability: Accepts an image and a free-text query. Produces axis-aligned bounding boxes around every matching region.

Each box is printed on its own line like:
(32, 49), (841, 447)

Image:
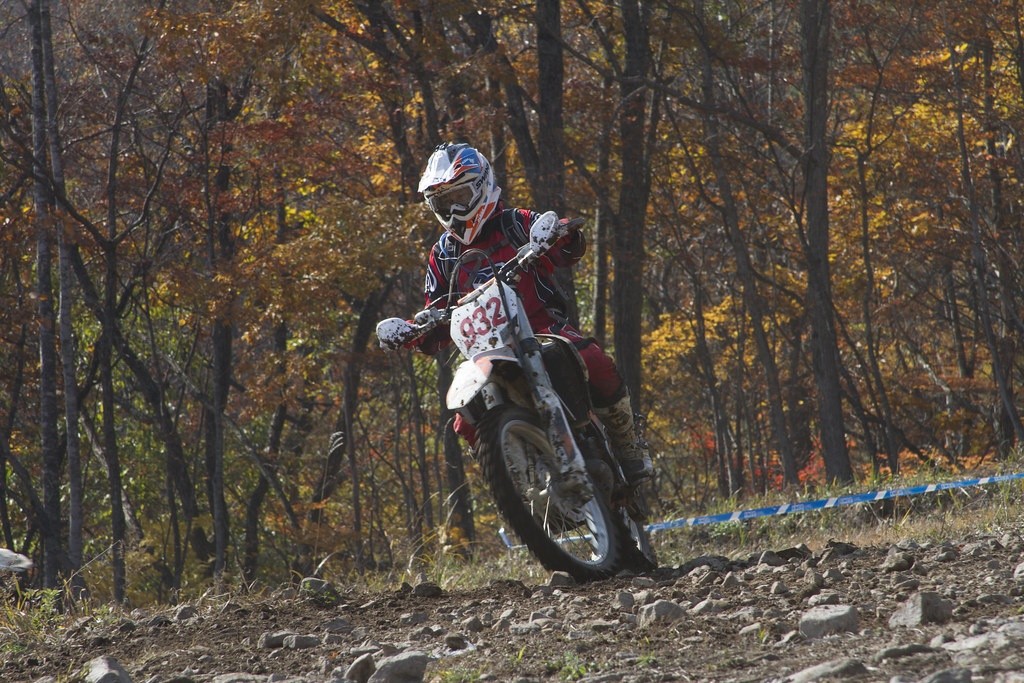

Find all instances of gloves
(376, 317), (423, 354)
(529, 210), (560, 257)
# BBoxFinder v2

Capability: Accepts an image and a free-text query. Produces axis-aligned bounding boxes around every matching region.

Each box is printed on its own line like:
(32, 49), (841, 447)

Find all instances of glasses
(425, 152), (490, 213)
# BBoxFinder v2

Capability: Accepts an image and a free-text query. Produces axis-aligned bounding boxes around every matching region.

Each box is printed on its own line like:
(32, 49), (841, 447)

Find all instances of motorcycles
(375, 211), (661, 584)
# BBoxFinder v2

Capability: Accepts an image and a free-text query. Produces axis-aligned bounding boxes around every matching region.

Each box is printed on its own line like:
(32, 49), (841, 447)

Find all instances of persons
(376, 140), (654, 479)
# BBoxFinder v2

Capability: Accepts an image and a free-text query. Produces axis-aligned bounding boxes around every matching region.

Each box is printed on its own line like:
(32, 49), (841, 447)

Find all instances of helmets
(417, 142), (502, 246)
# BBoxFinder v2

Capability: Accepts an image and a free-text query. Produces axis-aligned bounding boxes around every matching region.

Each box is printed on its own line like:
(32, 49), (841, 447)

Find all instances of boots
(592, 394), (652, 479)
